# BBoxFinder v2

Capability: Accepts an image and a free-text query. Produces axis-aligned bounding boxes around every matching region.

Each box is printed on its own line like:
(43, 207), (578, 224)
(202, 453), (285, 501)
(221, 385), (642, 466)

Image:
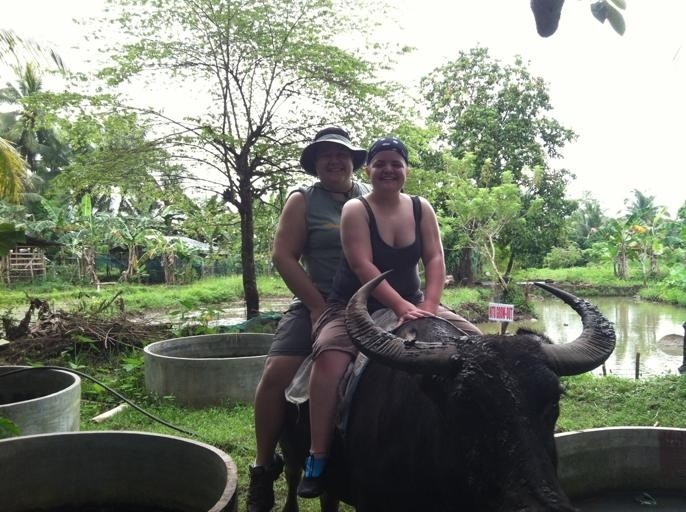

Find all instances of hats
(300, 132), (368, 176)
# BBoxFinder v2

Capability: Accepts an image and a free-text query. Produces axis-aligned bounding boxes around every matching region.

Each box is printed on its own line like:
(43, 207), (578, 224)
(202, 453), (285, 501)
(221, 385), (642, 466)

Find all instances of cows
(279, 269), (618, 512)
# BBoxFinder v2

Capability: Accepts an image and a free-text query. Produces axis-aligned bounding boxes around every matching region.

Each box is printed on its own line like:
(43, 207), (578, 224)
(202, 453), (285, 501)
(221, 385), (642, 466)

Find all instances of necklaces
(311, 180), (355, 200)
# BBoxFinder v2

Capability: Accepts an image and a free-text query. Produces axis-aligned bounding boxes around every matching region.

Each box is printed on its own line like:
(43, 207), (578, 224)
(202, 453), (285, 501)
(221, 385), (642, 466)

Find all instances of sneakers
(246, 452), (285, 512)
(297, 455), (338, 498)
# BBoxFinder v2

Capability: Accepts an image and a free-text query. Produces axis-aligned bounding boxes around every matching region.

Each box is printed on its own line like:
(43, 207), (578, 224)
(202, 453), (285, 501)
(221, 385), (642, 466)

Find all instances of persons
(295, 137), (481, 497)
(247, 127), (374, 511)
